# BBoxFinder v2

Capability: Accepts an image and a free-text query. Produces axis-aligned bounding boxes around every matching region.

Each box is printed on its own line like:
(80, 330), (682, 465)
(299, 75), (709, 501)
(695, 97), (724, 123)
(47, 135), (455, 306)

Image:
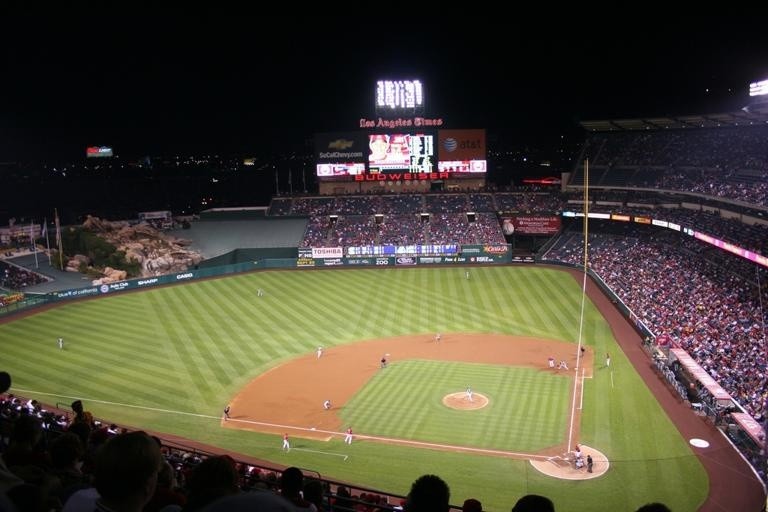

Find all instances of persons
(0, 333), (672, 512)
(0, 120), (565, 305)
(565, 121), (767, 487)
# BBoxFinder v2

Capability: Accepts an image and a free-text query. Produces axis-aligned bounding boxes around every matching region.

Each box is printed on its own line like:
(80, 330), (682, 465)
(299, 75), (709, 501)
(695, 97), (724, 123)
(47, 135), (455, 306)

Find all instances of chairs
(287, 111), (766, 486)
